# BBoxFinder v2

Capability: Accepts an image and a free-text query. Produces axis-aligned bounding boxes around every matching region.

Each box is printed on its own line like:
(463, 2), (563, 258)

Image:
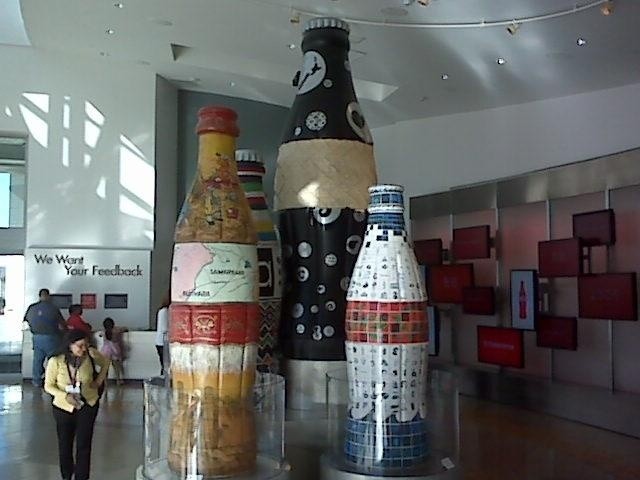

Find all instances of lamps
(288, 0), (614, 36)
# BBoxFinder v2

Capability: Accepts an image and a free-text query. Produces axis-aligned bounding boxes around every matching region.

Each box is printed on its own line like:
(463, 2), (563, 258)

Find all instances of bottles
(518, 280), (527, 321)
(272, 14), (379, 406)
(230, 146), (283, 414)
(165, 105), (261, 476)
(343, 185), (433, 468)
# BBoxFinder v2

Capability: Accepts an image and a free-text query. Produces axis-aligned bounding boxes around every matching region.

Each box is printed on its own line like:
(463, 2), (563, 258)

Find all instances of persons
(44, 329), (110, 480)
(24, 289), (74, 388)
(65, 304), (90, 336)
(156, 290), (170, 375)
(99, 318), (128, 389)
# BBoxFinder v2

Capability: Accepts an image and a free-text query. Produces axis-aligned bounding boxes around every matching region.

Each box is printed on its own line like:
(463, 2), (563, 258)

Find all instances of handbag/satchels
(93, 370), (104, 399)
